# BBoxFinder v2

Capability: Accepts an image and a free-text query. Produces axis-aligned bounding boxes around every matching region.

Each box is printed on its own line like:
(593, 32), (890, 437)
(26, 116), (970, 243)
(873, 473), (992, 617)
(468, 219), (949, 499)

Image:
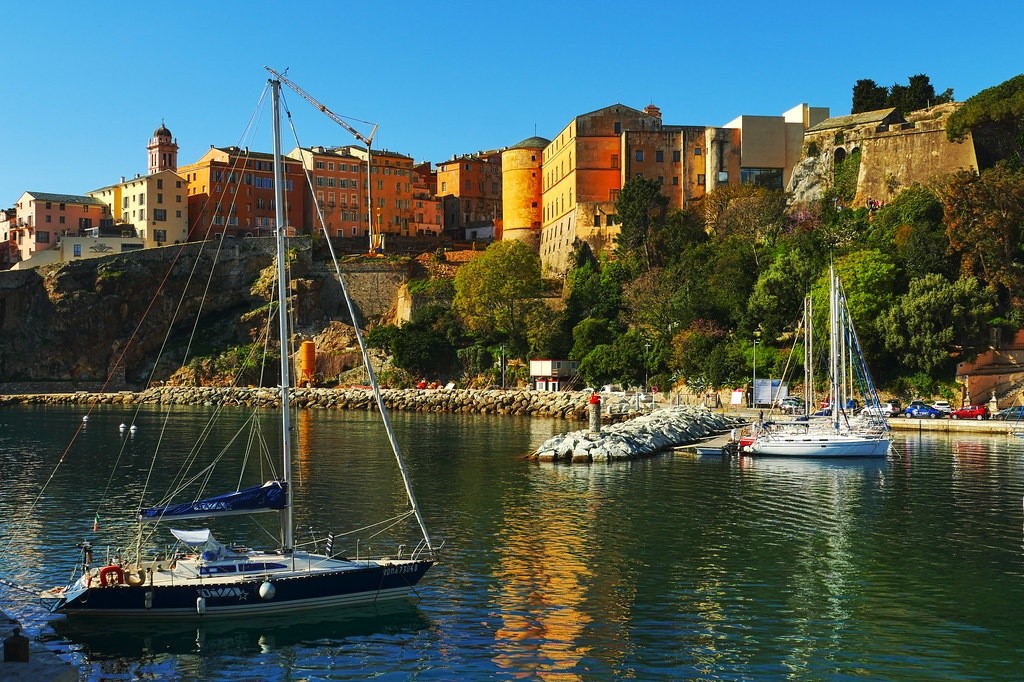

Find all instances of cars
(581, 388), (599, 395)
(993, 406), (1024, 421)
(632, 392), (653, 403)
(949, 405), (991, 421)
(904, 404), (943, 420)
(860, 402), (900, 418)
(777, 395), (805, 407)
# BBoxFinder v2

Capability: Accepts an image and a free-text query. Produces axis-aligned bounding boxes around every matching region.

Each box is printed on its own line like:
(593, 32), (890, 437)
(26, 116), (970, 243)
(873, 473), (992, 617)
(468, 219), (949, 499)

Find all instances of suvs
(931, 401), (951, 415)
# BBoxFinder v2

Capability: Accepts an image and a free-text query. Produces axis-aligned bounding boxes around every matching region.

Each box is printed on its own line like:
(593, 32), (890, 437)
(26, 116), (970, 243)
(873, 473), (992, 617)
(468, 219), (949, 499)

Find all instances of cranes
(263, 64), (380, 254)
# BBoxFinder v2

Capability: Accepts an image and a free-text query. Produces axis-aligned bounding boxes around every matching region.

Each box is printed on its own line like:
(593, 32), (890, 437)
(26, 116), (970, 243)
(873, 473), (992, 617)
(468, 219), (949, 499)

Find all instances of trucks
(597, 384), (636, 396)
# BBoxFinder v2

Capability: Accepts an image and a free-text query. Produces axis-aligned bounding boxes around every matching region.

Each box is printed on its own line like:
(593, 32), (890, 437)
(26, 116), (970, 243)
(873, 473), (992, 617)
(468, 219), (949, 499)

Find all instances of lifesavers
(125, 568), (146, 585)
(100, 566), (123, 586)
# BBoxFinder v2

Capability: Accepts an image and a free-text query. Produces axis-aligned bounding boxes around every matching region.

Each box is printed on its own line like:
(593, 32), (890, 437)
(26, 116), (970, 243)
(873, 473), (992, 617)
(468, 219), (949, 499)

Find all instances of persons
(447, 379), (458, 389)
(758, 409), (763, 426)
(417, 377), (428, 389)
(866, 197), (885, 208)
(430, 379), (442, 390)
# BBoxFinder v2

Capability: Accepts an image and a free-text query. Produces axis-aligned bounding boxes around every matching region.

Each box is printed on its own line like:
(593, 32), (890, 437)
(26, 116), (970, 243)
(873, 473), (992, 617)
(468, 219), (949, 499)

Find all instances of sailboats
(739, 249), (891, 458)
(0, 66), (444, 632)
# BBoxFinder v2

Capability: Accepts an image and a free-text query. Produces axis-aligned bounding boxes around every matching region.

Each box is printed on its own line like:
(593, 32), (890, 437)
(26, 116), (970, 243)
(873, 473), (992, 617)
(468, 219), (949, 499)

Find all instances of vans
(888, 399), (901, 417)
(910, 400), (924, 405)
